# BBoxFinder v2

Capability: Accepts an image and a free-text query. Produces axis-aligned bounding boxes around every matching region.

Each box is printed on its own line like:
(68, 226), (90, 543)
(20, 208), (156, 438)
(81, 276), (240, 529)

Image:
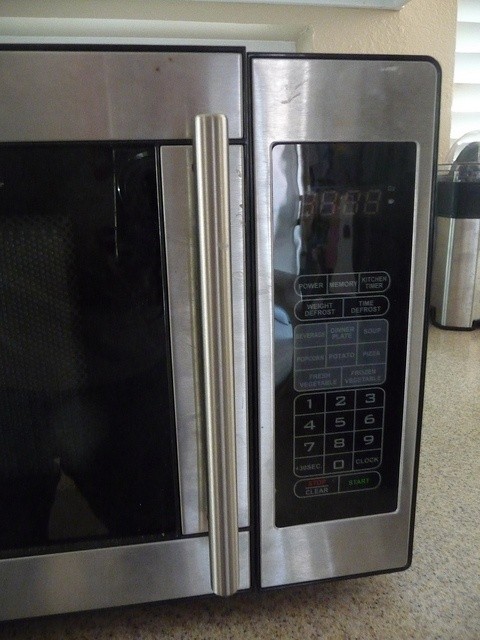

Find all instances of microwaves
(0, 42), (442, 628)
